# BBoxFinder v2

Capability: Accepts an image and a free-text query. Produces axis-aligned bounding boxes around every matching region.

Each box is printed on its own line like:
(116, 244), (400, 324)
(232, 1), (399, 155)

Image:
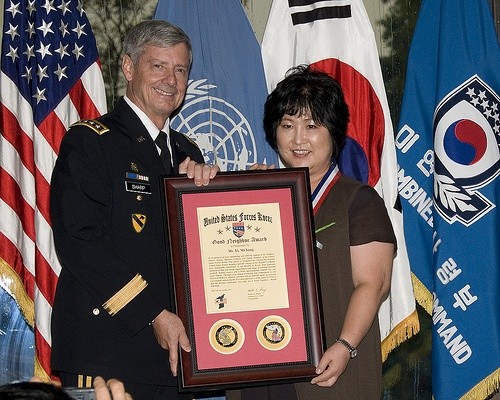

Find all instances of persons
(49, 20), (220, 388)
(225, 64), (398, 400)
(0, 376), (133, 400)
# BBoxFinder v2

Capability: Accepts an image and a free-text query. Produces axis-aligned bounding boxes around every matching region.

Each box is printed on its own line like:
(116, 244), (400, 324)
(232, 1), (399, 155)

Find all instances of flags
(0, 0), (107, 387)
(262, 0), (420, 364)
(152, 0), (281, 172)
(397, 0), (500, 400)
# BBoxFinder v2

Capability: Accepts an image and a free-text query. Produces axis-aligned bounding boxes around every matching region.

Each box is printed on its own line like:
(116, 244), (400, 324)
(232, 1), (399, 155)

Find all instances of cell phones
(62, 387), (113, 400)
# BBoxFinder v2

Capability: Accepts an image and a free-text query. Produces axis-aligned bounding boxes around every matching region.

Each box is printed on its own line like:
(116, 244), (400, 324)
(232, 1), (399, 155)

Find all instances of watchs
(336, 337), (358, 359)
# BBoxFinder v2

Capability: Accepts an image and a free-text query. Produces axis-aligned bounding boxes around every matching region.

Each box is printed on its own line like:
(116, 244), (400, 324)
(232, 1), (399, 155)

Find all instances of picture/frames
(158, 167), (328, 393)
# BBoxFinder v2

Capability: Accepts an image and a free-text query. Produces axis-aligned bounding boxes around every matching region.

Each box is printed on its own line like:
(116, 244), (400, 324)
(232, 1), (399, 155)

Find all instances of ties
(155, 131), (172, 176)
